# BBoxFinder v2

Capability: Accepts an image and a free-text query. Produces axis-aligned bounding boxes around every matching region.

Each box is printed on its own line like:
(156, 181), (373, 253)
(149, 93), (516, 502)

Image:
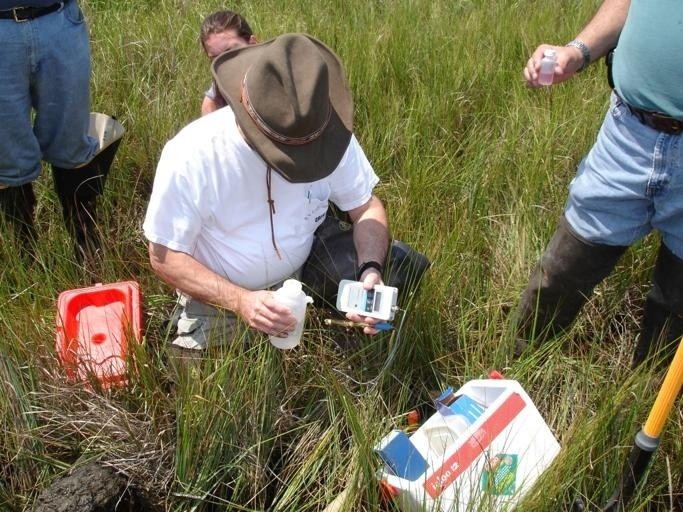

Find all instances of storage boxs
(50, 280), (142, 401)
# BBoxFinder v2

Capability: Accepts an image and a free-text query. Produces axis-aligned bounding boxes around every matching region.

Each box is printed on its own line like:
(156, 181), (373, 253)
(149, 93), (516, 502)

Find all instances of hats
(212, 32), (354, 183)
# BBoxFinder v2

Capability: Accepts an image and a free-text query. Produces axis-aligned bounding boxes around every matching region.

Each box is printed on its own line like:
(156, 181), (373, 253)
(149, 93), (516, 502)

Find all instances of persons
(143, 33), (431, 368)
(0, 1), (103, 264)
(511, 0), (683, 370)
(200, 11), (256, 118)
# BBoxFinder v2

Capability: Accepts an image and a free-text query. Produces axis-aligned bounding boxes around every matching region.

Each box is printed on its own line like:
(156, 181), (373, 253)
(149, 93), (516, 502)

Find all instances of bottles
(266, 279), (309, 351)
(535, 48), (558, 88)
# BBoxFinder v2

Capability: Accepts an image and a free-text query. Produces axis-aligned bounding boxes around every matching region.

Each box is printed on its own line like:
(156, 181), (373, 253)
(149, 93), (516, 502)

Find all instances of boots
(45, 116), (127, 267)
(4, 187), (42, 284)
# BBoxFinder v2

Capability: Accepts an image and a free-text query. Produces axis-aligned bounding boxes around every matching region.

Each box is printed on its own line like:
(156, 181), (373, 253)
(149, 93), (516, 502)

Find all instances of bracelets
(565, 41), (590, 72)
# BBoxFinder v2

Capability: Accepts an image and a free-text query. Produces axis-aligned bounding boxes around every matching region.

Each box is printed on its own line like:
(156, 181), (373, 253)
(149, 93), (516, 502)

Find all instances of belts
(607, 47), (681, 136)
(0, 0), (65, 22)
